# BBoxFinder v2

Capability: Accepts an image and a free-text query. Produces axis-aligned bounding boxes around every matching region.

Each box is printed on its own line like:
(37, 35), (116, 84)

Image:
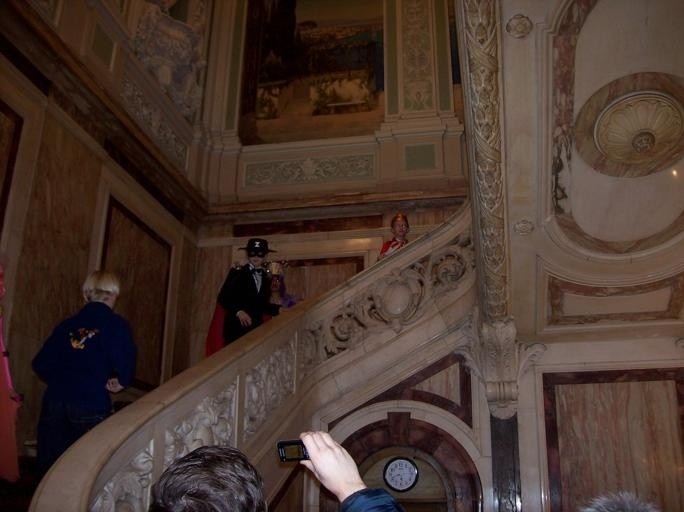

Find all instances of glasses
(248, 252), (265, 258)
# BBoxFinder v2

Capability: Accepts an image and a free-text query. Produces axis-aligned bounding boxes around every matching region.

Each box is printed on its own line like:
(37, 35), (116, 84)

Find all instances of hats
(238, 238), (277, 253)
(272, 263), (283, 275)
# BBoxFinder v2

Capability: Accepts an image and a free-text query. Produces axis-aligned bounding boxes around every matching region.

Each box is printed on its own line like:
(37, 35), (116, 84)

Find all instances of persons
(581, 490), (658, 512)
(0, 263), (24, 511)
(31, 269), (138, 479)
(377, 215), (410, 262)
(149, 429), (405, 512)
(203, 237), (304, 359)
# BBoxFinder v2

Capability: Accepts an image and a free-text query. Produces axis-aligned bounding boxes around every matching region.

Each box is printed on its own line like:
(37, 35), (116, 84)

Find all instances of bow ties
(251, 268), (262, 274)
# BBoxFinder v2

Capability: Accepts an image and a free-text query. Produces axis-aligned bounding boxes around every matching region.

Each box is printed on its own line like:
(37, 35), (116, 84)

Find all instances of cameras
(276, 440), (310, 463)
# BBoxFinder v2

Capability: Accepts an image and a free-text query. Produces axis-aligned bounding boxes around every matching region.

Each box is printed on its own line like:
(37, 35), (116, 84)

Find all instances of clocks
(383, 456), (419, 493)
